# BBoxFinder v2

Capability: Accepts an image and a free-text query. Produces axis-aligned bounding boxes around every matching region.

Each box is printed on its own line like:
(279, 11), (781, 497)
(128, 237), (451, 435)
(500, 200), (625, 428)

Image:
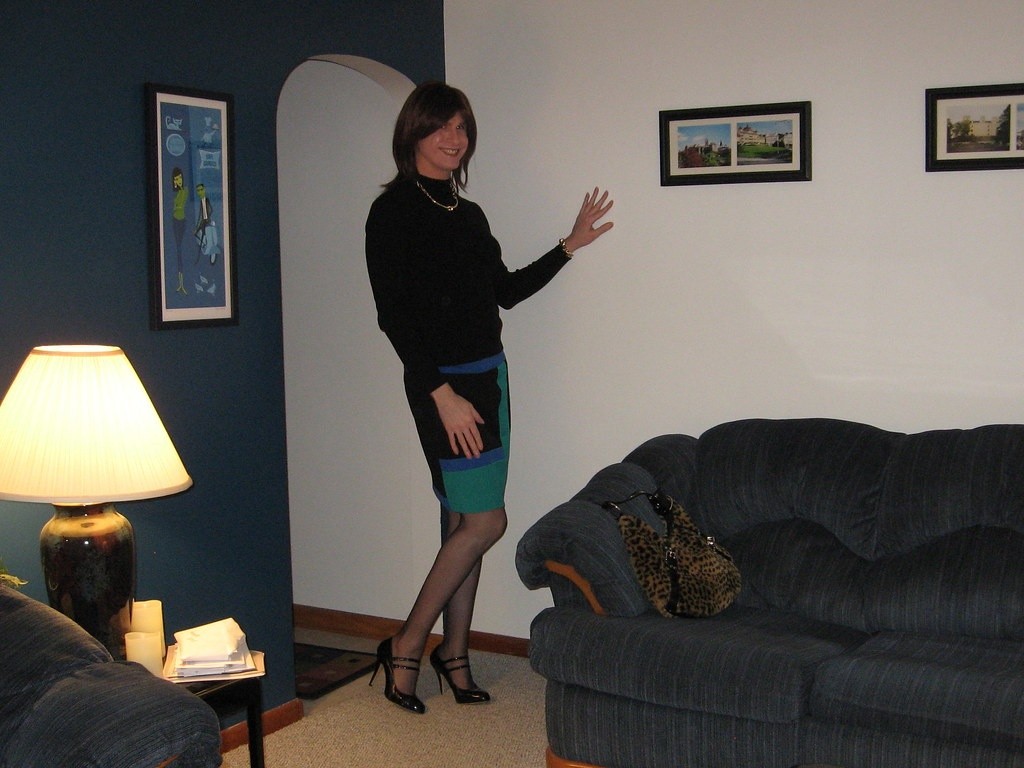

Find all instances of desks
(182, 649), (266, 768)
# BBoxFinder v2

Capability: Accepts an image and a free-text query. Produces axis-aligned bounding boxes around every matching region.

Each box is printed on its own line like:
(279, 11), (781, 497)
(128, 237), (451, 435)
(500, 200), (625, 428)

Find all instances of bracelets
(559, 238), (574, 259)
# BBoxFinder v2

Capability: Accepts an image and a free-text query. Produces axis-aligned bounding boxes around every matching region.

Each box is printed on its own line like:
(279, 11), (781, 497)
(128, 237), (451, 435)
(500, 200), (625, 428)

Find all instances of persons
(365, 86), (615, 713)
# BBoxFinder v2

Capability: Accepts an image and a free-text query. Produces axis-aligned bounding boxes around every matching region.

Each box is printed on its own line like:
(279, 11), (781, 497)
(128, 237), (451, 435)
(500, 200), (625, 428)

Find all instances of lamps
(0, 344), (193, 663)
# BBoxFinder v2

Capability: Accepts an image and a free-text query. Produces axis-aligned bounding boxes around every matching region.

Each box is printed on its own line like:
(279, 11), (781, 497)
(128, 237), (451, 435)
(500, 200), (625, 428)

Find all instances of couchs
(514, 419), (1024, 768)
(0, 583), (222, 768)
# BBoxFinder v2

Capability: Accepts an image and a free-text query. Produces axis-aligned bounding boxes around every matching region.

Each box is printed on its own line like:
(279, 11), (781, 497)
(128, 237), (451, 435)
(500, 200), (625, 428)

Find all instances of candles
(130, 599), (166, 658)
(126, 632), (163, 678)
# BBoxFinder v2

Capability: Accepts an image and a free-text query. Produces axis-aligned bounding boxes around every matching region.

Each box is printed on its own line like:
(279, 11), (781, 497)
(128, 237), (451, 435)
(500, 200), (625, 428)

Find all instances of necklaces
(416, 180), (459, 211)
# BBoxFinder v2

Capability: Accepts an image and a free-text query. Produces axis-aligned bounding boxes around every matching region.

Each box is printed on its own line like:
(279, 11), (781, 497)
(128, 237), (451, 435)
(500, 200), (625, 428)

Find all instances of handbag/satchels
(621, 499), (741, 620)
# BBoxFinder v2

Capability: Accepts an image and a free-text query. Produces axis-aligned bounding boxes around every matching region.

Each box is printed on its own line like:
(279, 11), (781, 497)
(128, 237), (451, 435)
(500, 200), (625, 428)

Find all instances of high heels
(429, 647), (490, 705)
(369, 637), (427, 713)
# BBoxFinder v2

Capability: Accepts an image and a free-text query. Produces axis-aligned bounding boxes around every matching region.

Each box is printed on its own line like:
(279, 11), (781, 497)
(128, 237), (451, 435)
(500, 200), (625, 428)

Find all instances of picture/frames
(659, 101), (813, 186)
(925, 83), (1024, 172)
(145, 83), (239, 330)
